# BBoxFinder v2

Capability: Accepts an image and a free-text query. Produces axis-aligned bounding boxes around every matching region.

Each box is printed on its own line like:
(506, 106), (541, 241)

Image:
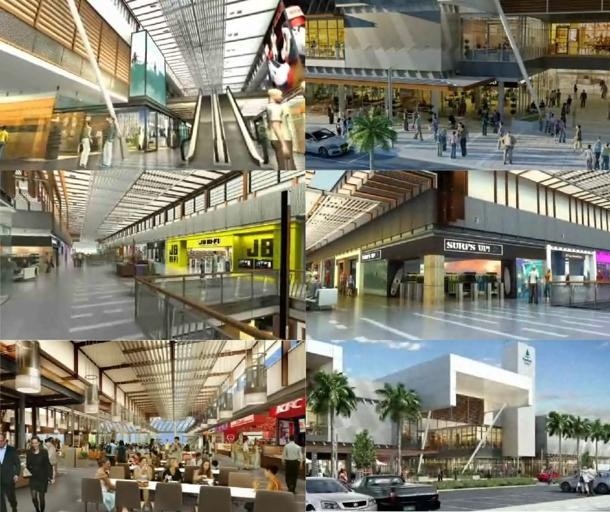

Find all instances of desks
(109, 478), (257, 502)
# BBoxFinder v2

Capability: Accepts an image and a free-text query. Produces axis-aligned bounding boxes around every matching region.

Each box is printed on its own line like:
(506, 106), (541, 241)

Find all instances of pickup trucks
(355, 475), (440, 510)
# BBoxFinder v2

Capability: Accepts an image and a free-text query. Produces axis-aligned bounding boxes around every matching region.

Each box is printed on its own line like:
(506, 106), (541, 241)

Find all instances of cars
(560, 469), (610, 494)
(305, 475), (378, 511)
(306, 125), (350, 159)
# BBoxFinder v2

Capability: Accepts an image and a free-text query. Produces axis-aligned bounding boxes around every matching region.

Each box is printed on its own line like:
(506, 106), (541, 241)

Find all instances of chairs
(154, 484), (183, 512)
(81, 478), (102, 512)
(149, 465), (155, 480)
(115, 479), (141, 512)
(218, 467), (237, 485)
(185, 466), (199, 483)
(254, 490), (299, 512)
(194, 486), (232, 512)
(228, 472), (249, 486)
(109, 466), (125, 479)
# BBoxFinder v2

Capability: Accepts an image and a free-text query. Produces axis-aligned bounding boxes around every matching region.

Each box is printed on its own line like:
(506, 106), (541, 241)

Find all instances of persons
(454, 467), (459, 480)
(547, 466), (598, 496)
(101, 118), (116, 167)
(441, 470), (443, 481)
(0, 126), (8, 158)
(306, 267), (354, 296)
(545, 269), (551, 297)
(339, 469), (348, 483)
(313, 37), (608, 169)
(437, 467), (440, 481)
(2, 429), (304, 510)
(264, 87), (296, 169)
(528, 265), (539, 305)
(79, 117), (93, 169)
